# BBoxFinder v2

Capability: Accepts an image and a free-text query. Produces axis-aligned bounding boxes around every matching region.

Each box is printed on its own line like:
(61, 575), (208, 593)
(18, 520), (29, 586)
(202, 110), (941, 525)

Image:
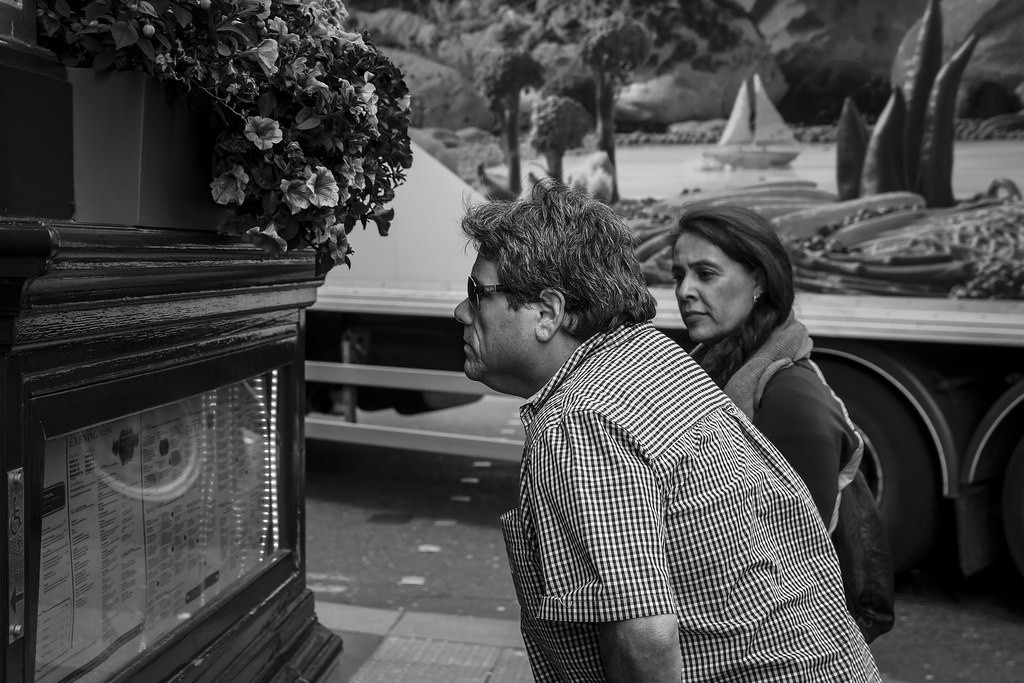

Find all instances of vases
(62, 66), (243, 233)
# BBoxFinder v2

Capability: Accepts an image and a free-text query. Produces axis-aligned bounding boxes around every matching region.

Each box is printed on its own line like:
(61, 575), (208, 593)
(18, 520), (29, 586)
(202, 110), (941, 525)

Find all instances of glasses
(466, 276), (526, 313)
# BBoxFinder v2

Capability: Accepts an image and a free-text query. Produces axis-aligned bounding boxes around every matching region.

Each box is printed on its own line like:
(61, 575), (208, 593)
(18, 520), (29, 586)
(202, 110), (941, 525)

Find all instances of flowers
(36, 0), (413, 274)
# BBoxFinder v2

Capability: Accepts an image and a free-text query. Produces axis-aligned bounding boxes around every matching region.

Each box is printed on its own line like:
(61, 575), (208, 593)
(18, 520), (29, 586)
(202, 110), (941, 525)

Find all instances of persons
(454, 180), (880, 683)
(671, 206), (896, 645)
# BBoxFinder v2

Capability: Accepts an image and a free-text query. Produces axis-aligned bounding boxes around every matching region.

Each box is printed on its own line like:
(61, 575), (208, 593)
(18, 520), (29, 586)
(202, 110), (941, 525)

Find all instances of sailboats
(701, 73), (801, 171)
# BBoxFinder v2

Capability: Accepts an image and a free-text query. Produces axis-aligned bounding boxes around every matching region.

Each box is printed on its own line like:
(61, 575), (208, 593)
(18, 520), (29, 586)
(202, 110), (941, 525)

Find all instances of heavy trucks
(304, 140), (1023, 623)
